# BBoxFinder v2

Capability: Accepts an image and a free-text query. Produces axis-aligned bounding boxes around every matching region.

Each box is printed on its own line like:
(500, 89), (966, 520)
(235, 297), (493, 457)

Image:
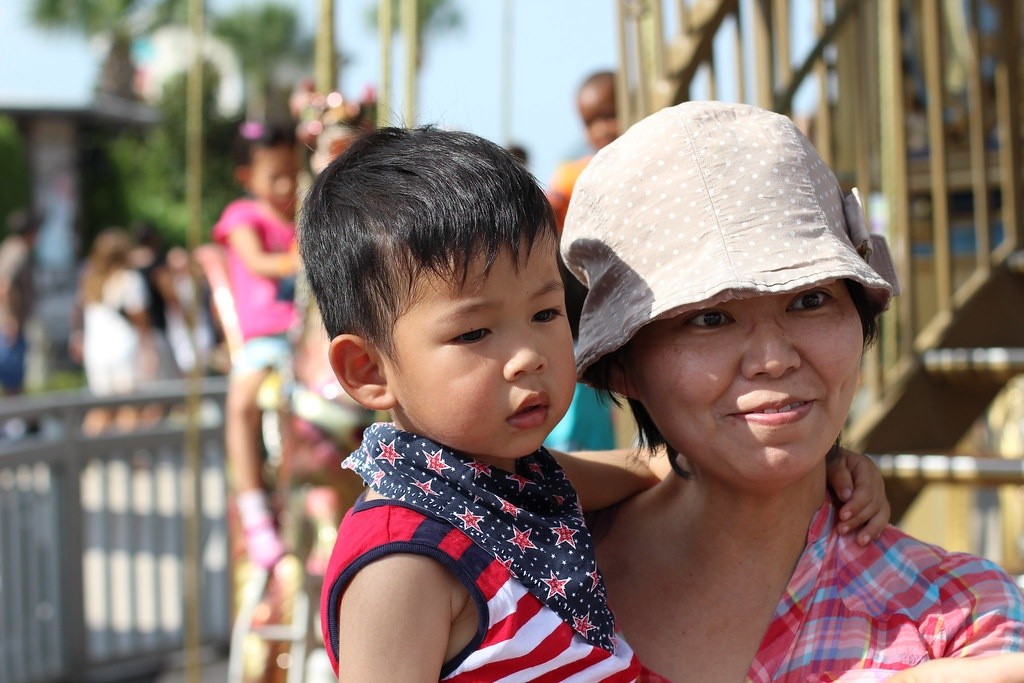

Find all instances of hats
(559, 100), (901, 382)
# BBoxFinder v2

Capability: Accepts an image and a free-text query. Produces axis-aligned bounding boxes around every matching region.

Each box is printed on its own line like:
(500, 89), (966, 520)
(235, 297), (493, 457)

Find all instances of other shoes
(305, 546), (335, 575)
(238, 510), (283, 571)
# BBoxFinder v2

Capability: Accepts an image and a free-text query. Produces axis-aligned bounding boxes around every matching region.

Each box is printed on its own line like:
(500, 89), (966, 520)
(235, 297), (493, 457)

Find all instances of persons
(225, 83), (380, 683)
(208, 118), (312, 562)
(0, 198), (238, 441)
(541, 70), (621, 230)
(561, 97), (1023, 683)
(298, 123), (893, 680)
